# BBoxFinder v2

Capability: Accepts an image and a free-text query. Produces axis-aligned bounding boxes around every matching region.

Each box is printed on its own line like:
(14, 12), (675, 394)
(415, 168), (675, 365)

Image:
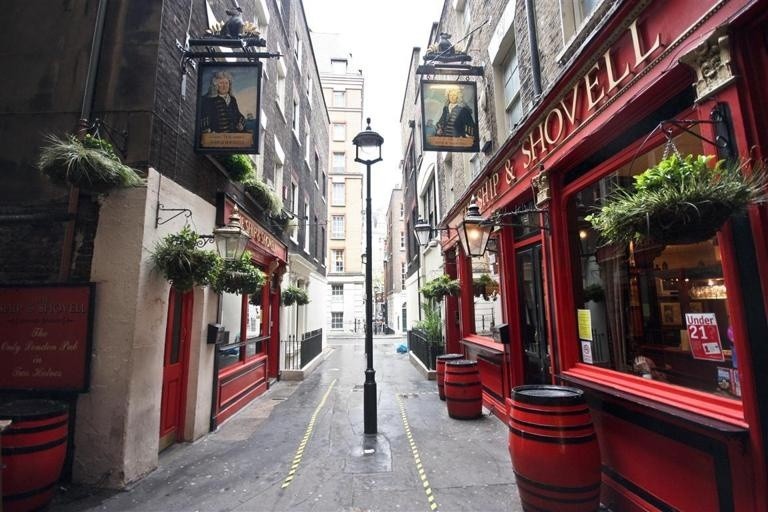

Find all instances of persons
(633, 355), (661, 380)
(201, 70), (247, 132)
(434, 85), (475, 136)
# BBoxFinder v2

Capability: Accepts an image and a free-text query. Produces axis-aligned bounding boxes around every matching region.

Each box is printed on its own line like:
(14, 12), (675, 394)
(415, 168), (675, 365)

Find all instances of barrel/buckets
(507, 384), (602, 512)
(444, 359), (482, 420)
(436, 353), (464, 401)
(0, 398), (71, 512)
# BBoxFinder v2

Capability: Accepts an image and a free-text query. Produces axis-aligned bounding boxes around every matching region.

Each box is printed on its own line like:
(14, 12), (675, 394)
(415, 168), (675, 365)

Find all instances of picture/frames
(419, 79), (480, 154)
(193, 61), (263, 155)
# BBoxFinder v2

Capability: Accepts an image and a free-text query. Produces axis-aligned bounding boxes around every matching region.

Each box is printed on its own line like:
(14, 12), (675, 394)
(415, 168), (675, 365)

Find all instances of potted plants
(143, 218), (312, 307)
(34, 127), (147, 195)
(582, 151), (767, 264)
(471, 272), (500, 302)
(417, 272), (463, 303)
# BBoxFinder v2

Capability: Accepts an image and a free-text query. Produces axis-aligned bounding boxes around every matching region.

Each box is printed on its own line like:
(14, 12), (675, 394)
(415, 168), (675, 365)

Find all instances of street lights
(352, 115), (388, 438)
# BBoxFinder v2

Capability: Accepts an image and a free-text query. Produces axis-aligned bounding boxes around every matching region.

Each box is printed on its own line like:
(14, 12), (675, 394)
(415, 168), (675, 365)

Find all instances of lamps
(455, 202), (552, 258)
(154, 202), (252, 262)
(412, 215), (456, 247)
